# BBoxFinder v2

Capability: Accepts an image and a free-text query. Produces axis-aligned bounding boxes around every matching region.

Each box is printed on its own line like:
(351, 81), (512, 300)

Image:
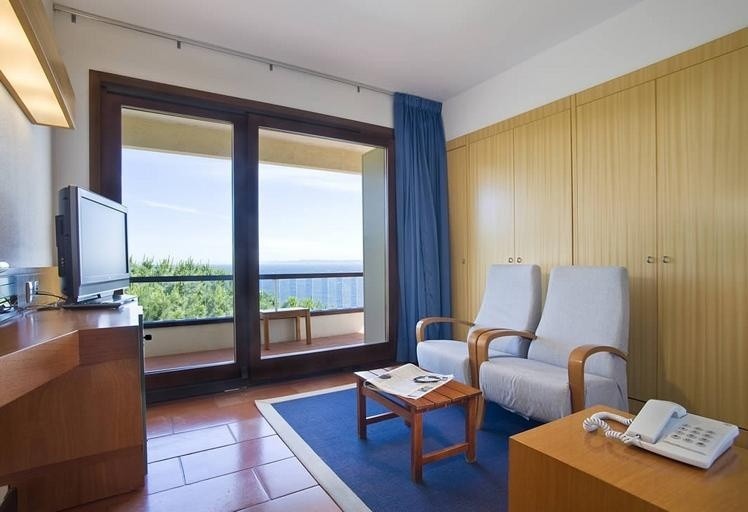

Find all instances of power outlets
(27, 281), (33, 303)
(33, 281), (39, 295)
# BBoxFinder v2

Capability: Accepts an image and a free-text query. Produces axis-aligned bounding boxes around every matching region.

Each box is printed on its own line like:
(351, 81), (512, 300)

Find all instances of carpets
(254, 383), (546, 512)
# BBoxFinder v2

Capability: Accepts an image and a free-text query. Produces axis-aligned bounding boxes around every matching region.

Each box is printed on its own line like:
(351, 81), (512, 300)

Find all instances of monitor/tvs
(54, 184), (138, 309)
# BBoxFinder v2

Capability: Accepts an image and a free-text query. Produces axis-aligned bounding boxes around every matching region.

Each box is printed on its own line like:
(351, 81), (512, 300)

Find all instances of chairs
(475, 265), (631, 431)
(415, 263), (541, 429)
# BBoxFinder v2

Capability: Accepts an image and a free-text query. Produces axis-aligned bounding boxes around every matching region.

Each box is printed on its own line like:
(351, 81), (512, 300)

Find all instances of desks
(507, 404), (748, 512)
(260, 307), (312, 350)
(0, 294), (152, 512)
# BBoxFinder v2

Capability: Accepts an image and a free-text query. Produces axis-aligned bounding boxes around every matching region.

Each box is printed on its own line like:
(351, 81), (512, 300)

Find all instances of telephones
(624, 399), (739, 470)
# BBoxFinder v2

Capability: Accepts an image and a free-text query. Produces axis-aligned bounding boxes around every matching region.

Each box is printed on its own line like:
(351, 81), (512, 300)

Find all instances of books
(364, 363), (456, 400)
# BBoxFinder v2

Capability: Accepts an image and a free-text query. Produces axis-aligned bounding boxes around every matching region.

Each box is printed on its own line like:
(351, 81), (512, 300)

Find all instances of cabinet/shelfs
(445, 96), (572, 344)
(572, 26), (748, 429)
